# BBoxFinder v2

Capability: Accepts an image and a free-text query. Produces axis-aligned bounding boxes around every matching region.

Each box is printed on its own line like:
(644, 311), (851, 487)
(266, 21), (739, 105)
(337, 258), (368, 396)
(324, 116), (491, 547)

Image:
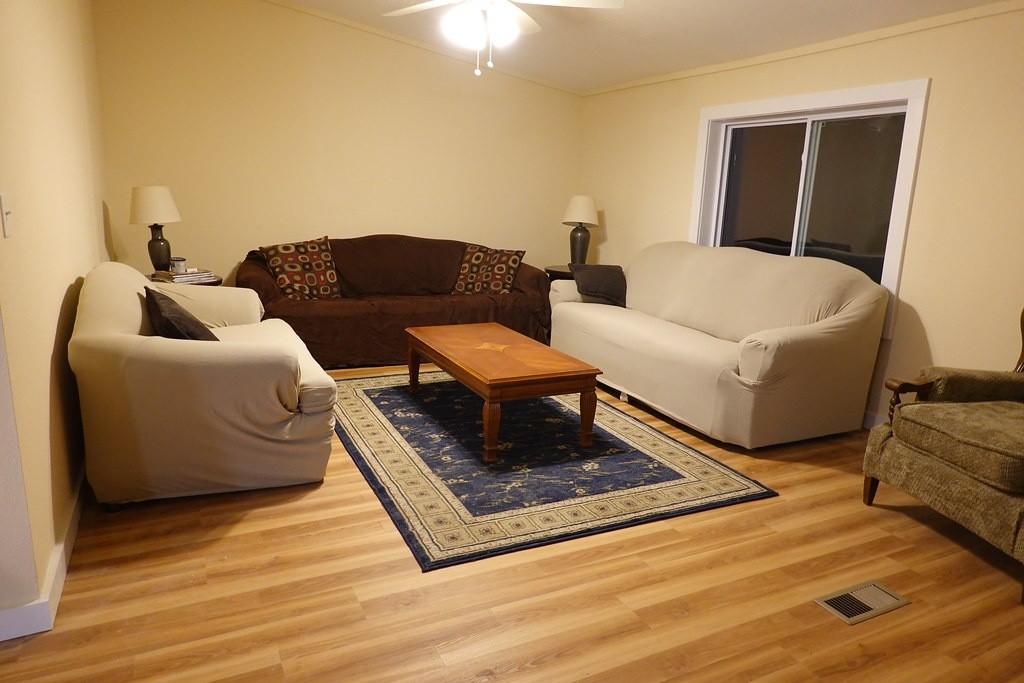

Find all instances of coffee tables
(401, 323), (604, 459)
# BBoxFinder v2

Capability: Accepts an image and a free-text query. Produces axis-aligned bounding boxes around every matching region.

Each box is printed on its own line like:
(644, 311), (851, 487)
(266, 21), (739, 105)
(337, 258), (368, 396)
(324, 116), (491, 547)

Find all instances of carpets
(332, 367), (780, 573)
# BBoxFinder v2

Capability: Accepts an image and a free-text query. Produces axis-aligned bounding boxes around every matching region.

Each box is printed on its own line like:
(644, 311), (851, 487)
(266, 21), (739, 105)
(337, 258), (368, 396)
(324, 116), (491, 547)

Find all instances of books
(155, 268), (217, 283)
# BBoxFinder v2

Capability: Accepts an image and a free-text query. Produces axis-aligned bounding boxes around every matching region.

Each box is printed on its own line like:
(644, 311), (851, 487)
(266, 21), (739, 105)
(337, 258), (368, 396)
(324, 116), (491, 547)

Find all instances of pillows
(258, 236), (342, 300)
(143, 285), (219, 342)
(567, 263), (627, 307)
(451, 244), (526, 295)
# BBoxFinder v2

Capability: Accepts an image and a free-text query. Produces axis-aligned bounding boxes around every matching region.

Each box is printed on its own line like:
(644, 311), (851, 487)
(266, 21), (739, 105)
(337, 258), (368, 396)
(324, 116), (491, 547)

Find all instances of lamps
(437, 3), (522, 77)
(129, 184), (185, 279)
(561, 193), (602, 262)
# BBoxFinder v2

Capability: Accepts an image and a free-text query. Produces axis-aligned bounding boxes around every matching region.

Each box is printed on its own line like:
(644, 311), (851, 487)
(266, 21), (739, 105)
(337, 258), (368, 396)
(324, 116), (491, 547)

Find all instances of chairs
(862, 309), (1024, 602)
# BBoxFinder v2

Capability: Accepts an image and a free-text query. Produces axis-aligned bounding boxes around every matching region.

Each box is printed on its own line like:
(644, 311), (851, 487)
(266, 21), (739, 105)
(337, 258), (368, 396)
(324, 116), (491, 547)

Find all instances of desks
(140, 270), (221, 284)
(544, 264), (577, 281)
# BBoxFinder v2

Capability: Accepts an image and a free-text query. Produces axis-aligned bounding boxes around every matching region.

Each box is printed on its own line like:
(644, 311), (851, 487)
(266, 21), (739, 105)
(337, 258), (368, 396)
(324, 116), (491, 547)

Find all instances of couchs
(66, 260), (338, 511)
(236, 234), (553, 369)
(549, 240), (889, 452)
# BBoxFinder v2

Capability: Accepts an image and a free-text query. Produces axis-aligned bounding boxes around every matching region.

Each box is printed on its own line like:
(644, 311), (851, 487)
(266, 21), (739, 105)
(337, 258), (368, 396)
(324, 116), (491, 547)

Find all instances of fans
(383, 1), (625, 38)
